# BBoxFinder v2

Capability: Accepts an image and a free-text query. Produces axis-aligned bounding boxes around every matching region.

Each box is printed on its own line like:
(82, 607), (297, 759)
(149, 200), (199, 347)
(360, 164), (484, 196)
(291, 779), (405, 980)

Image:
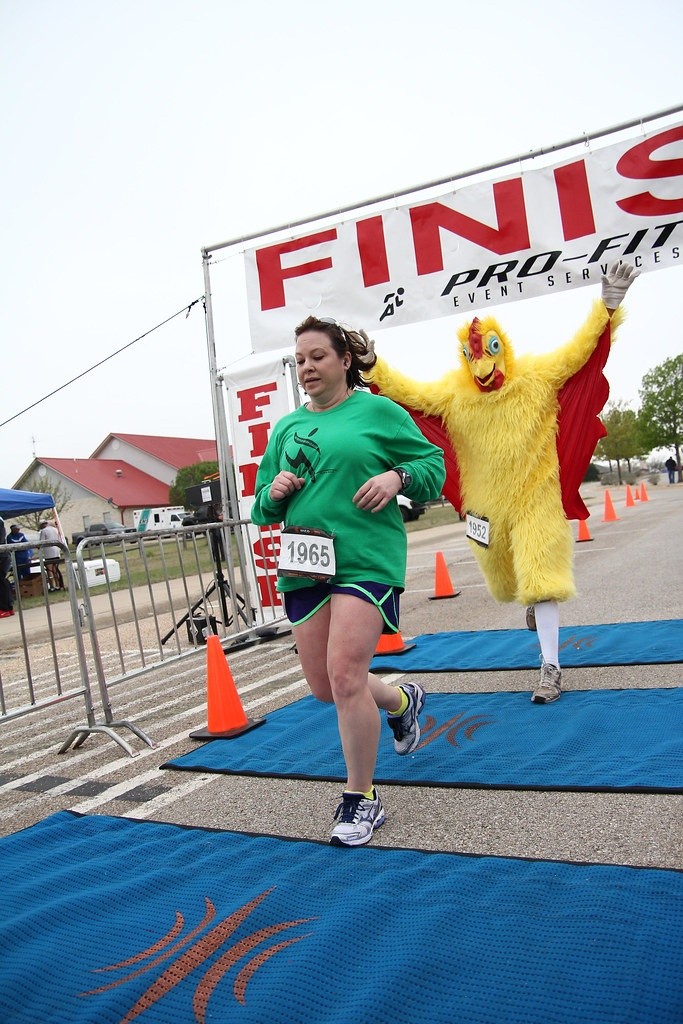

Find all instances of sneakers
(525, 604), (538, 631)
(530, 660), (562, 704)
(329, 785), (386, 847)
(385, 682), (425, 755)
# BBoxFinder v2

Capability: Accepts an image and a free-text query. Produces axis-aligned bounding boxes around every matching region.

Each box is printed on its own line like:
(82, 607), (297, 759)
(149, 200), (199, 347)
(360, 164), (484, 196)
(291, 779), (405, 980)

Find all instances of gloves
(347, 330), (375, 365)
(599, 261), (643, 310)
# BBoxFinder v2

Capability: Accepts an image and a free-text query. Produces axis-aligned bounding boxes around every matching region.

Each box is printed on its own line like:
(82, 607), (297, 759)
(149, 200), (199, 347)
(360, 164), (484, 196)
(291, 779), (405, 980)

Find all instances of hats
(11, 525), (22, 529)
(39, 519), (47, 526)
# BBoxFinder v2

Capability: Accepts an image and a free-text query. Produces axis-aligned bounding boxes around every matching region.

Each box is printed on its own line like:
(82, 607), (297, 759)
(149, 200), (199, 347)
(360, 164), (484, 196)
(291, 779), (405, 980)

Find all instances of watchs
(394, 468), (411, 491)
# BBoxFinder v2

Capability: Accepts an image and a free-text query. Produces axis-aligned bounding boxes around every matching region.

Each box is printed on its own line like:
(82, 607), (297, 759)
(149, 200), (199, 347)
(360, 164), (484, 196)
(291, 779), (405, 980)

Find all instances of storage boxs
(72, 559), (121, 589)
(19, 573), (44, 598)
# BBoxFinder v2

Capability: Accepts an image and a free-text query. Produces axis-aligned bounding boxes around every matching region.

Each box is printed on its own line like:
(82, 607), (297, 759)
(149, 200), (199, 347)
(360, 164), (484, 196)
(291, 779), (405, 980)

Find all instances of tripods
(160, 503), (256, 645)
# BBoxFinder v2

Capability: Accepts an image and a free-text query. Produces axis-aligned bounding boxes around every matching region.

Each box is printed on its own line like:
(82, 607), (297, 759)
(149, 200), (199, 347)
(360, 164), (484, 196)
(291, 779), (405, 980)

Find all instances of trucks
(133, 506), (202, 538)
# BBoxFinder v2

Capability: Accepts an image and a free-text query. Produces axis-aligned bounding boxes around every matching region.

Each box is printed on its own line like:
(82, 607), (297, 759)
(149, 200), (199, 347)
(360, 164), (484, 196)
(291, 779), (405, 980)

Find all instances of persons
(249, 313), (446, 848)
(37, 519), (60, 592)
(0, 516), (15, 618)
(665, 457), (676, 483)
(348, 261), (641, 703)
(7, 524), (33, 579)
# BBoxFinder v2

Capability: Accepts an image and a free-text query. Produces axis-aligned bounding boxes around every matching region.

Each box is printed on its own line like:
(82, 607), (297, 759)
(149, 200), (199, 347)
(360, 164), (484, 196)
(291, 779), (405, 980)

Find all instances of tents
(0, 488), (80, 589)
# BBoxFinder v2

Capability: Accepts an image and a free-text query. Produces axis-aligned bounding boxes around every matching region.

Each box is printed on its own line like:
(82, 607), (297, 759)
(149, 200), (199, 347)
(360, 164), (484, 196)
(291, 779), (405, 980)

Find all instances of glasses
(318, 317), (346, 341)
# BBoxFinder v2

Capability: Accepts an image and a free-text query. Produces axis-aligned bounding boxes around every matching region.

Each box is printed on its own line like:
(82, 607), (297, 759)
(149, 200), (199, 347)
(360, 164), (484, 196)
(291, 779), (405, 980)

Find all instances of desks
(7, 558), (66, 591)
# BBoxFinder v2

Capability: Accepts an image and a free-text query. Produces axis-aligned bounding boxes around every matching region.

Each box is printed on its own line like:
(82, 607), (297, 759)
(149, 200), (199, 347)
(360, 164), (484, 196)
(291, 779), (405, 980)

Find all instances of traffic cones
(374, 630), (417, 655)
(624, 485), (638, 507)
(189, 635), (267, 740)
(640, 482), (649, 502)
(634, 488), (640, 500)
(427, 551), (462, 600)
(601, 490), (621, 522)
(575, 519), (594, 543)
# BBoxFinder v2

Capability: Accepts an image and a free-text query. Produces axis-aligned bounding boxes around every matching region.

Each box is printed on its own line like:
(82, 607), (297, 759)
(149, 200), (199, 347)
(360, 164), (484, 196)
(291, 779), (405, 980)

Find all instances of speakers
(185, 477), (231, 507)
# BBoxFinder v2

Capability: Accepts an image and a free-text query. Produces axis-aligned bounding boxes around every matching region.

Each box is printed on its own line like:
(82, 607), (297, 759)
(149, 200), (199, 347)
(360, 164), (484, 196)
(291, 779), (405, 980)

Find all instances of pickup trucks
(71, 521), (137, 548)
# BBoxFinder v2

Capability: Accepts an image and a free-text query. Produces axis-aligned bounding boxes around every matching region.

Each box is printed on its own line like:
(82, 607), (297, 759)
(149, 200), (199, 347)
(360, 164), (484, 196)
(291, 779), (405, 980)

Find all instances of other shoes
(48, 587), (60, 592)
(0, 607), (14, 618)
